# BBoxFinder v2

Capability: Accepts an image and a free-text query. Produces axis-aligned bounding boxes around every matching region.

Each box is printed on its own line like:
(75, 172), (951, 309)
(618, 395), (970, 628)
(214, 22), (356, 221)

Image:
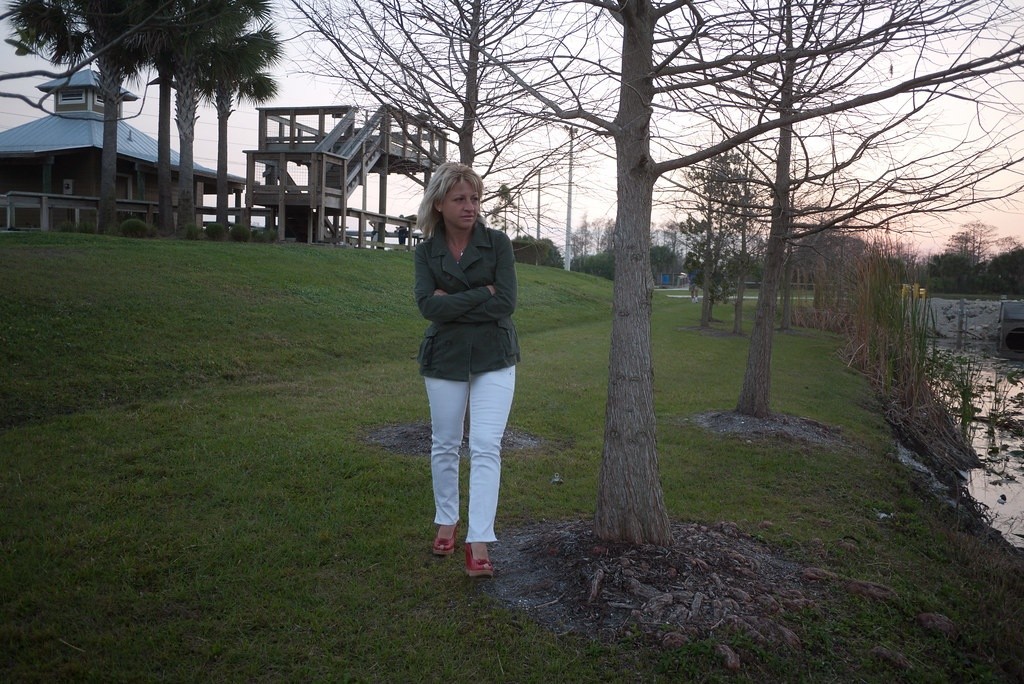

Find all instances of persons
(413, 162), (521, 579)
(369, 221), (387, 249)
(394, 215), (407, 246)
(687, 269), (698, 304)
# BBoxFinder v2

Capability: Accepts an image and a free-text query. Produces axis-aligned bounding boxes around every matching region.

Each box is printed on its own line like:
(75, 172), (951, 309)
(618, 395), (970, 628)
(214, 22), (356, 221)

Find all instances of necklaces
(451, 246), (466, 257)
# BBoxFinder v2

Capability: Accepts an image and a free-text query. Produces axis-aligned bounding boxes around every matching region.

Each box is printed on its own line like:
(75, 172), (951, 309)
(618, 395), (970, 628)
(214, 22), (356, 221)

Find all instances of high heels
(464, 543), (493, 577)
(434, 523), (459, 554)
